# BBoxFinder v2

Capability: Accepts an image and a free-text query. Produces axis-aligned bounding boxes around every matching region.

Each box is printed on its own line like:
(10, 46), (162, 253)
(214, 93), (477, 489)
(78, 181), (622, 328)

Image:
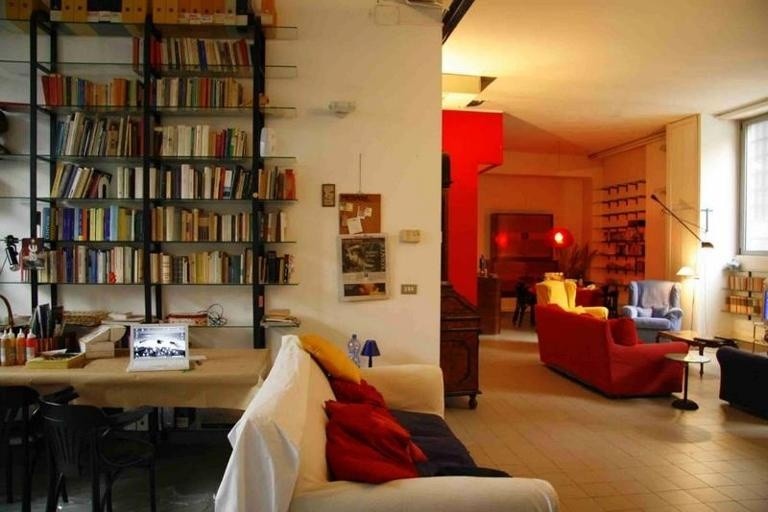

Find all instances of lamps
(1, 235), (20, 274)
(651, 194), (715, 248)
(360, 340), (380, 367)
(675, 267), (699, 329)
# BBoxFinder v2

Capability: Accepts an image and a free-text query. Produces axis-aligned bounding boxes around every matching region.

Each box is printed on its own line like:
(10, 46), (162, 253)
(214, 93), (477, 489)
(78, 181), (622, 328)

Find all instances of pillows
(298, 333), (362, 383)
(651, 304), (668, 318)
(636, 306), (653, 318)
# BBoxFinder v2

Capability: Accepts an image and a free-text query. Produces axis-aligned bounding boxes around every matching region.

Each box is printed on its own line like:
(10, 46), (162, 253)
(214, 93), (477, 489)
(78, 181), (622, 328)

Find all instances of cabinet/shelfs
(592, 177), (645, 291)
(0, 1), (300, 451)
(721, 270), (764, 319)
(490, 212), (560, 294)
(441, 282), (483, 409)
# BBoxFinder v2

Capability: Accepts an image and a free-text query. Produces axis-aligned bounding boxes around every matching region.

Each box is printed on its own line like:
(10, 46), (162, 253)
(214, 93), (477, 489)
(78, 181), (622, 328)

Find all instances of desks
(0, 346), (271, 510)
(478, 273), (502, 334)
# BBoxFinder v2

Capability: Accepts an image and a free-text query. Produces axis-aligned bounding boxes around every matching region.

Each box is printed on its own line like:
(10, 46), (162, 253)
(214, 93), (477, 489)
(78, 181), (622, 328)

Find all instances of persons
(348, 245), (375, 270)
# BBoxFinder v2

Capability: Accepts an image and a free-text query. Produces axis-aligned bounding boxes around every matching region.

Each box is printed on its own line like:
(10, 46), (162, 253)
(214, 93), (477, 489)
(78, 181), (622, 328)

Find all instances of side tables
(664, 352), (710, 411)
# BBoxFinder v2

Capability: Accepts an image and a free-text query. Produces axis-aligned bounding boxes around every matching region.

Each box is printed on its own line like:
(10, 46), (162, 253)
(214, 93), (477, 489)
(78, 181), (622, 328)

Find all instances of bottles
(348, 333), (361, 368)
(0, 328), (37, 367)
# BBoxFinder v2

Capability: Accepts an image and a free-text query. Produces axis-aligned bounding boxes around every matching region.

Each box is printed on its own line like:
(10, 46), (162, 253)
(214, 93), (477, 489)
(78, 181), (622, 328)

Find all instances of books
(37, 75), (147, 284)
(133, 36), (253, 77)
(258, 166), (299, 284)
(727, 273), (766, 316)
(149, 75), (255, 284)
(20, 249), (32, 283)
(101, 308), (300, 328)
(6, 0), (277, 39)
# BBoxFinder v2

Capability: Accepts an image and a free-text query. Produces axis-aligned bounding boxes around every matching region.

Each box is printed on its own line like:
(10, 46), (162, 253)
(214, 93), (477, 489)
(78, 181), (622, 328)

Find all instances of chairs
(512, 282), (537, 328)
(0, 385), (159, 510)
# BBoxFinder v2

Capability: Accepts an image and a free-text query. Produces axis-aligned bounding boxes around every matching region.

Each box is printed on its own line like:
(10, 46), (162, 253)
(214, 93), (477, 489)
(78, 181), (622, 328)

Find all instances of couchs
(535, 279), (609, 320)
(622, 279), (683, 343)
(716, 345), (768, 421)
(535, 302), (689, 396)
(214, 329), (559, 511)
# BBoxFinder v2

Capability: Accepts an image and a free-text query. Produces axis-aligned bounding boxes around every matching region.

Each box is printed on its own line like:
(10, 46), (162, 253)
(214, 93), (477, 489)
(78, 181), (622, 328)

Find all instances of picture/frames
(336, 234), (391, 302)
(321, 183), (336, 207)
